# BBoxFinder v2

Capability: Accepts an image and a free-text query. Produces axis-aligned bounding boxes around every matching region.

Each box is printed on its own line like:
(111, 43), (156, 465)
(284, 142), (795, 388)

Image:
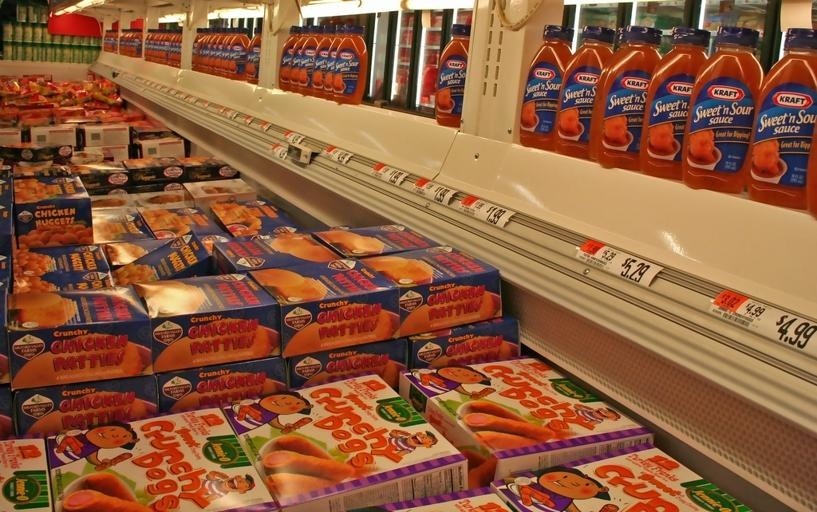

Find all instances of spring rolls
(261, 436), (352, 498)
(63, 471), (157, 510)
(461, 396), (556, 452)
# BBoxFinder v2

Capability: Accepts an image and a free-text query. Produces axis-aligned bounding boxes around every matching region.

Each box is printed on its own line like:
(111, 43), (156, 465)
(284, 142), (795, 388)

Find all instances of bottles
(599, 27), (665, 169)
(683, 26), (764, 200)
(641, 25), (712, 185)
(748, 26), (816, 215)
(514, 26), (575, 153)
(279, 23), (367, 105)
(104, 28), (182, 69)
(192, 28), (262, 85)
(558, 26), (615, 161)
(430, 22), (469, 129)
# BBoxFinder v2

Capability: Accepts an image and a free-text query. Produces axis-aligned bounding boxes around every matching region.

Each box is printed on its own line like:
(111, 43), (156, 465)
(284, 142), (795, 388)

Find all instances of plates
(457, 400), (490, 433)
(55, 470), (137, 511)
(257, 435), (331, 479)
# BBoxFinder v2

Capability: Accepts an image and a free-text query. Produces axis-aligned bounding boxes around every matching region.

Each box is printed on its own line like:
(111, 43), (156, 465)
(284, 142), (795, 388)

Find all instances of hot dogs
(1, 353), (9, 378)
(429, 334), (520, 371)
(157, 317), (280, 373)
(170, 372), (287, 415)
(24, 390), (157, 437)
(300, 354), (406, 392)
(10, 331), (152, 389)
(0, 415), (12, 438)
(395, 283), (500, 338)
(283, 302), (401, 357)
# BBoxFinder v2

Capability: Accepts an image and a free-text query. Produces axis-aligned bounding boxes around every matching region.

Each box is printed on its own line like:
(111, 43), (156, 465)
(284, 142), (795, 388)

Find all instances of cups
(435, 98), (456, 114)
